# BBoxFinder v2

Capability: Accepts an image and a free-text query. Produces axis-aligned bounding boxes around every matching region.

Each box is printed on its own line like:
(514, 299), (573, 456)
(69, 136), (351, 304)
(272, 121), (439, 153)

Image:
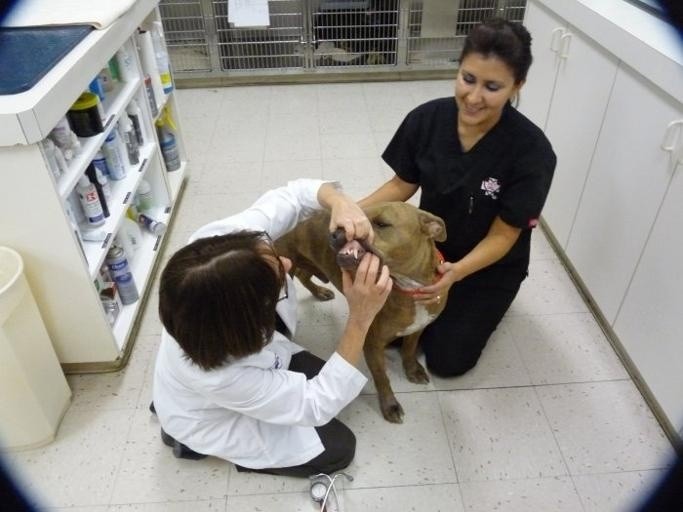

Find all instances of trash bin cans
(0, 246), (73, 453)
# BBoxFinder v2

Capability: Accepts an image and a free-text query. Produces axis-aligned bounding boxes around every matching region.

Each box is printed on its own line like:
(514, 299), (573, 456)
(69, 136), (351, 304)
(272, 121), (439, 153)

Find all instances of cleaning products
(155, 104), (181, 171)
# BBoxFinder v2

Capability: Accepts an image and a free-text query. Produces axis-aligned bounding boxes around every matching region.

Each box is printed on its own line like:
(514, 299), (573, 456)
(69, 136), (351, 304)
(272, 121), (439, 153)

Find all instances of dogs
(272, 201), (448, 424)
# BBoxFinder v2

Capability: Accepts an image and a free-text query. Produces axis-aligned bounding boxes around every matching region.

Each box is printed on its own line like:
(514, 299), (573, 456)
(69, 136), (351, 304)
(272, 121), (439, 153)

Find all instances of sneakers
(160, 426), (195, 460)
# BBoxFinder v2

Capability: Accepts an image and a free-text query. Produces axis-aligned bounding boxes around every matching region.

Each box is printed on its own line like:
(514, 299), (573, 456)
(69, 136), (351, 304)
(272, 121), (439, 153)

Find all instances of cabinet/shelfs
(0, 0), (189, 375)
(502, 0), (683, 457)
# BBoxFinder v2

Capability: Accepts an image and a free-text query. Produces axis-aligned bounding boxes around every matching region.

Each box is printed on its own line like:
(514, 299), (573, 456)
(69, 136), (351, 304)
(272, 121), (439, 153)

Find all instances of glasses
(259, 231), (289, 302)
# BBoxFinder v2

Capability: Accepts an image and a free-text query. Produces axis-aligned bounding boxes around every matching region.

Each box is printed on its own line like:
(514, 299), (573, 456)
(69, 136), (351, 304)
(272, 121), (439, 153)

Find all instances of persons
(149, 179), (393, 478)
(355, 18), (556, 378)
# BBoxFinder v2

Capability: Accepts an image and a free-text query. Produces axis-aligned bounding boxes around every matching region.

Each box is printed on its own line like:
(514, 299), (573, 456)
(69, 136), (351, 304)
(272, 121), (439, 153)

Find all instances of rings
(437, 296), (441, 305)
